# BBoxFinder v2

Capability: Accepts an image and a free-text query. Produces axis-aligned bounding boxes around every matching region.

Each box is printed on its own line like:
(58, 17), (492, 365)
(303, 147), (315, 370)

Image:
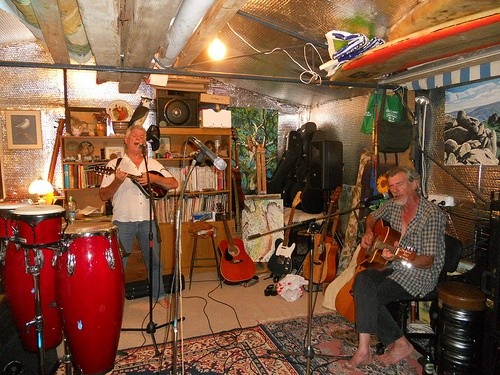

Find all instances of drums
(8, 204), (67, 248)
(0, 202), (33, 242)
(53, 219), (125, 375)
(1, 239), (63, 356)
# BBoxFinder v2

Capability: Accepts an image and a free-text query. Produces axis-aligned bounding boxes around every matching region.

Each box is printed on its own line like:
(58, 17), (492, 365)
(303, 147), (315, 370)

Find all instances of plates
(107, 101), (133, 122)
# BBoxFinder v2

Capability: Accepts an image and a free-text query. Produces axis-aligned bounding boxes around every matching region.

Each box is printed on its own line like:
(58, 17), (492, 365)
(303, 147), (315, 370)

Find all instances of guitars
(93, 165), (168, 200)
(215, 202), (256, 285)
(298, 187), (342, 285)
(335, 265), (365, 324)
(266, 191), (303, 275)
(356, 217), (417, 269)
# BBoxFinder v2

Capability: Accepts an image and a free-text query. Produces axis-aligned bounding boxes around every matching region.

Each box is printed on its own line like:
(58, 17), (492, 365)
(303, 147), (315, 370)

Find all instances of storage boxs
(198, 92), (233, 128)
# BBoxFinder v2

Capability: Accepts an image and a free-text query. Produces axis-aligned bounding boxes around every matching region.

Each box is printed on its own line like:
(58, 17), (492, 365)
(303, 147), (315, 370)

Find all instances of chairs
(401, 229), (462, 334)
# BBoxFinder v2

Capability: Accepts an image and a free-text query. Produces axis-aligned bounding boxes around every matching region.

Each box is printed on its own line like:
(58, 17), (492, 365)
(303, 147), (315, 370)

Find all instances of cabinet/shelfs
(57, 133), (234, 272)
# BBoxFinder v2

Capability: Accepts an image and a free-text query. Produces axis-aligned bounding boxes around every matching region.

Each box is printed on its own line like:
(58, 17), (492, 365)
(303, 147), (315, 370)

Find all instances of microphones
(365, 193), (389, 203)
(190, 136), (227, 170)
(138, 143), (147, 155)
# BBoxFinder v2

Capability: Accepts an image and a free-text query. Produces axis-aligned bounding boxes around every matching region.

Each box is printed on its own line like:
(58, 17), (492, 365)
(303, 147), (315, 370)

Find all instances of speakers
(156, 89), (199, 128)
(309, 140), (343, 192)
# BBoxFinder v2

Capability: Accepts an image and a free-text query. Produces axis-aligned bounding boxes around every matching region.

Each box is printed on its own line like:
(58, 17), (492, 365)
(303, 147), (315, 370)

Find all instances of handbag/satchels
(360, 84), (412, 153)
(277, 274), (309, 302)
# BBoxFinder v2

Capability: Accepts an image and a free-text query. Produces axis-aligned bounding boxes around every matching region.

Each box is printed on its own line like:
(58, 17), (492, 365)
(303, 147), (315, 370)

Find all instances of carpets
(55, 307), (430, 374)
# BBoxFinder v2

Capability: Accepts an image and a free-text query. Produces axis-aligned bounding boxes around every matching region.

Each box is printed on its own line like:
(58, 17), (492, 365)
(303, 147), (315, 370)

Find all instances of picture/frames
(67, 105), (107, 137)
(5, 109), (43, 149)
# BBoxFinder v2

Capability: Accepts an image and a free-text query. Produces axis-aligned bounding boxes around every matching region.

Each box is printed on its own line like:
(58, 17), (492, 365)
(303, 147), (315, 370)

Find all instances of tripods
(246, 201), (370, 375)
(121, 153), (185, 355)
(50, 334), (82, 375)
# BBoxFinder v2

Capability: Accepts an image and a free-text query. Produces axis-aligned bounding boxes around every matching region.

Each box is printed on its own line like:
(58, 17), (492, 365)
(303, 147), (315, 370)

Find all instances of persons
(99, 126), (178, 308)
(341, 166), (445, 368)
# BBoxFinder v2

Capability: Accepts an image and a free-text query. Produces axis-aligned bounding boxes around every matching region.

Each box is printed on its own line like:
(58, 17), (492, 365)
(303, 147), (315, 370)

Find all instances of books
(153, 165), (229, 223)
(65, 165), (105, 189)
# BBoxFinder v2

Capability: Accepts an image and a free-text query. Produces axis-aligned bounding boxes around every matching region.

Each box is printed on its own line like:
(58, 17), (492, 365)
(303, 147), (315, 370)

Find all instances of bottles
(423, 345), (434, 375)
(68, 196), (76, 223)
